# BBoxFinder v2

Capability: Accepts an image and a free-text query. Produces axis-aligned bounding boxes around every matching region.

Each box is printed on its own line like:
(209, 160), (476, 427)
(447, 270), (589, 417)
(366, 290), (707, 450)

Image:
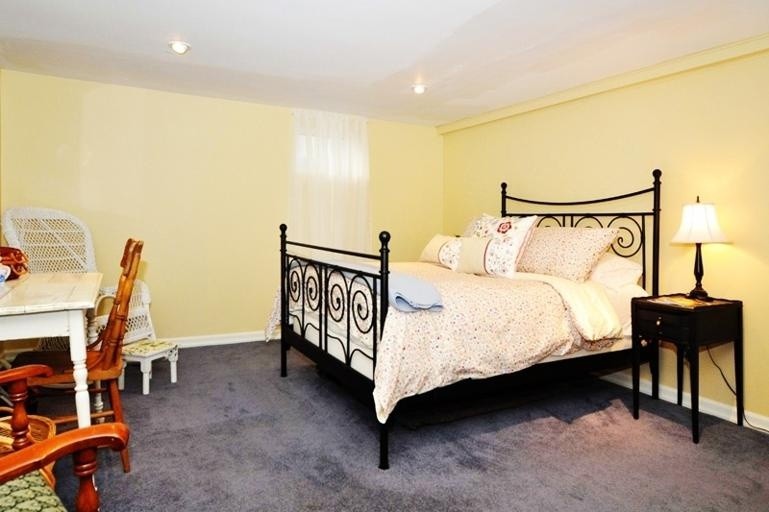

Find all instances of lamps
(671, 195), (732, 301)
(412, 83), (428, 95)
(168, 40), (191, 55)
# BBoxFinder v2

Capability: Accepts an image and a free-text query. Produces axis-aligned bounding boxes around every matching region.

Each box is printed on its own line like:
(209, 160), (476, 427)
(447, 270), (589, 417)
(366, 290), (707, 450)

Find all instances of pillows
(419, 212), (642, 292)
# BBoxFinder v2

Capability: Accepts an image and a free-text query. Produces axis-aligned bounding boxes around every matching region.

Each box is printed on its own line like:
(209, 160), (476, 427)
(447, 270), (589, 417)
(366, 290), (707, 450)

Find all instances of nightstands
(630, 293), (745, 444)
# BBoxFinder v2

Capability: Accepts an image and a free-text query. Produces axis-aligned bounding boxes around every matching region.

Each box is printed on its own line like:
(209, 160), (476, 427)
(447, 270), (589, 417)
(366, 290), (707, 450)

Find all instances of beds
(278, 169), (662, 471)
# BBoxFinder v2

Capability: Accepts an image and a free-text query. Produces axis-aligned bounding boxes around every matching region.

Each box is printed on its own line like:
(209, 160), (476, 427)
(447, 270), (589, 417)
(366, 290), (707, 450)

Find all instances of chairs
(25, 237), (145, 472)
(1, 364), (131, 512)
(0, 206), (179, 395)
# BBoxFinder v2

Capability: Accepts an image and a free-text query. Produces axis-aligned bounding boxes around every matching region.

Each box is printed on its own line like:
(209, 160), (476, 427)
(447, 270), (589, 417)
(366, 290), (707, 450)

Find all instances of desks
(1, 271), (104, 491)
